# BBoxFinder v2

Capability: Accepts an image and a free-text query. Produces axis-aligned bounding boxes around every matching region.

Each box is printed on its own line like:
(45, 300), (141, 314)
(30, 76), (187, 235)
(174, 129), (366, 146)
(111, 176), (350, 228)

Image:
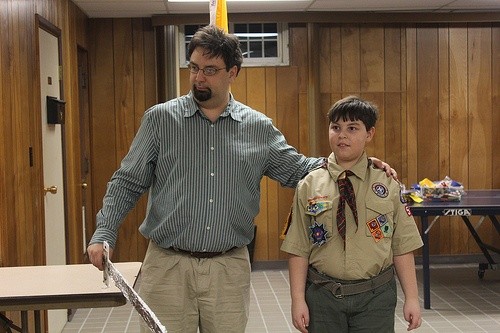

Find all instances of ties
(333, 170), (359, 250)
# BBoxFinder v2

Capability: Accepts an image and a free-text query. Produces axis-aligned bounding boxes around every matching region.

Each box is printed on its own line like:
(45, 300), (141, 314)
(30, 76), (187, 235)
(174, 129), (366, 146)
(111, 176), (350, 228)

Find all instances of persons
(85, 25), (403, 332)
(280, 93), (427, 333)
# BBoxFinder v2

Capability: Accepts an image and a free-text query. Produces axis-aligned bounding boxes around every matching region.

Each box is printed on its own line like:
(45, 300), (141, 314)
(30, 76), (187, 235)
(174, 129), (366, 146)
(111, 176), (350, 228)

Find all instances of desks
(405, 189), (500, 310)
(0, 261), (143, 333)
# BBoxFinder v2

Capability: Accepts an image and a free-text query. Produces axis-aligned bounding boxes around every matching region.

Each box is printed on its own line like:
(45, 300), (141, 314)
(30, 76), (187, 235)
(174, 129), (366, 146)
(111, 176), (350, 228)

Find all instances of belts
(162, 245), (238, 258)
(306, 267), (396, 297)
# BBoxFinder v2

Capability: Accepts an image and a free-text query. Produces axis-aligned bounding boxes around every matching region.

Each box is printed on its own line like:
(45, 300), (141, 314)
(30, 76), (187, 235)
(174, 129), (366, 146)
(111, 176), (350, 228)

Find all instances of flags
(208, 0), (230, 36)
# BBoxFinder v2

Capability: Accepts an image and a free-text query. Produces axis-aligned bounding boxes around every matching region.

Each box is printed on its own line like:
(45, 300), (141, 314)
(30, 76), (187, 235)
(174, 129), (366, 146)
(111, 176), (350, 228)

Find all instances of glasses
(187, 63), (231, 75)
